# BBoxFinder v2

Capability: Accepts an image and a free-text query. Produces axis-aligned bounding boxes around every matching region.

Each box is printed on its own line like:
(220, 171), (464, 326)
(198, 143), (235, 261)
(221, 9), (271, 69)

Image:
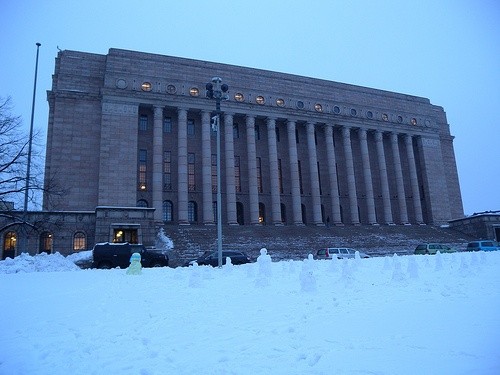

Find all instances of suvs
(91, 242), (169, 269)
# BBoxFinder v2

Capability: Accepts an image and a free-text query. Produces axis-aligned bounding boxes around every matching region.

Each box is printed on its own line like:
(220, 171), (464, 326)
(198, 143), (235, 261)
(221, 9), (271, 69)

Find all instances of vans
(413, 243), (458, 255)
(465, 240), (500, 252)
(313, 247), (370, 262)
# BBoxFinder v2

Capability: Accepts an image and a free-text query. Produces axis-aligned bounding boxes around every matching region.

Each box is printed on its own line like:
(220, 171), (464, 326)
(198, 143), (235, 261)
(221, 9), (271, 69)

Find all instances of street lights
(206, 76), (231, 268)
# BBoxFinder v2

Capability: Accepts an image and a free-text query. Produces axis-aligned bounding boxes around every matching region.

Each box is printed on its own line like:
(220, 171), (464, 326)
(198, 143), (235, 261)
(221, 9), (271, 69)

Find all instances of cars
(184, 249), (251, 267)
